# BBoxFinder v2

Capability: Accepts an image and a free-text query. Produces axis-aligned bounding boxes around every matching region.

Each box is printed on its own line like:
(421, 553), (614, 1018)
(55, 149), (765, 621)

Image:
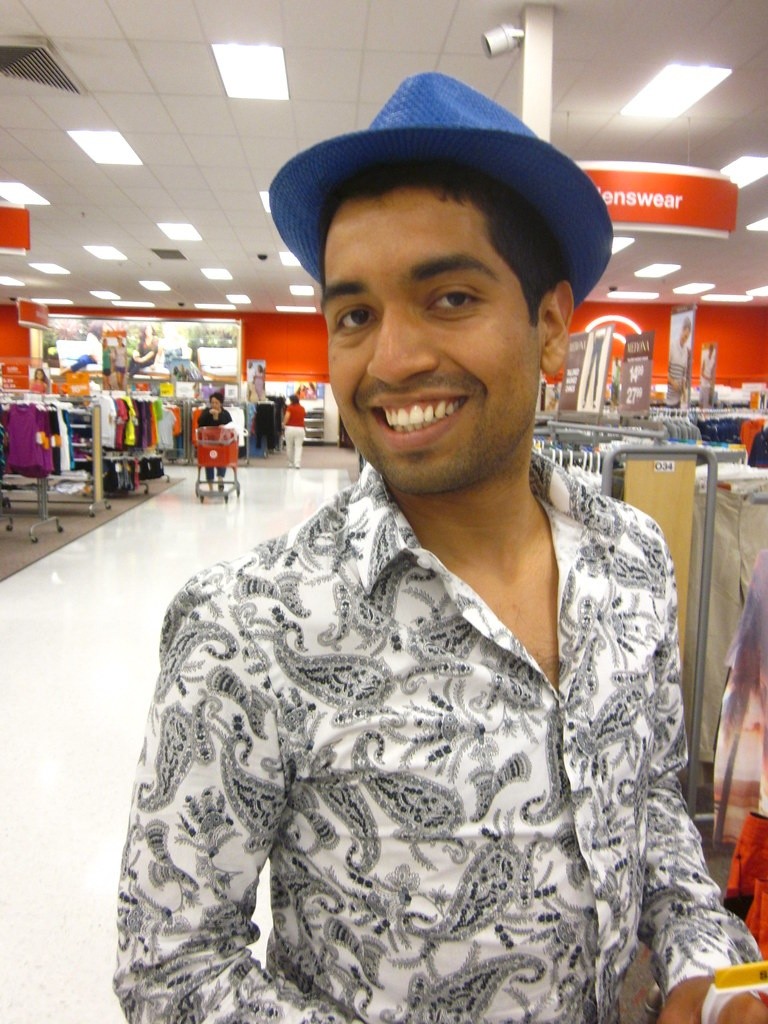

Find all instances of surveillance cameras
(178, 303), (184, 306)
(609, 286), (617, 291)
(10, 297), (16, 301)
(258, 254), (268, 260)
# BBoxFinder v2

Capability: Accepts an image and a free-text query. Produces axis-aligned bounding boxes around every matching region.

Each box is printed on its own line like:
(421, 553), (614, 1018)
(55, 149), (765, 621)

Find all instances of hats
(270, 72), (612, 307)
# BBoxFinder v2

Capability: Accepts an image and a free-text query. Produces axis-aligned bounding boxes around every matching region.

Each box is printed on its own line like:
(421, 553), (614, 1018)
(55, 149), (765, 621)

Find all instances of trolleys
(195, 425), (241, 505)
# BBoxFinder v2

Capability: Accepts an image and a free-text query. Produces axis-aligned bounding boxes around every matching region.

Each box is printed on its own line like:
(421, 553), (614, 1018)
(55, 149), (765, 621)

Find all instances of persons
(295, 381), (317, 400)
(246, 360), (265, 402)
(60, 320), (189, 392)
(111, 68), (767, 1023)
(666, 315), (691, 408)
(281, 394), (306, 469)
(197, 392), (232, 493)
(28, 368), (50, 392)
(701, 343), (715, 409)
